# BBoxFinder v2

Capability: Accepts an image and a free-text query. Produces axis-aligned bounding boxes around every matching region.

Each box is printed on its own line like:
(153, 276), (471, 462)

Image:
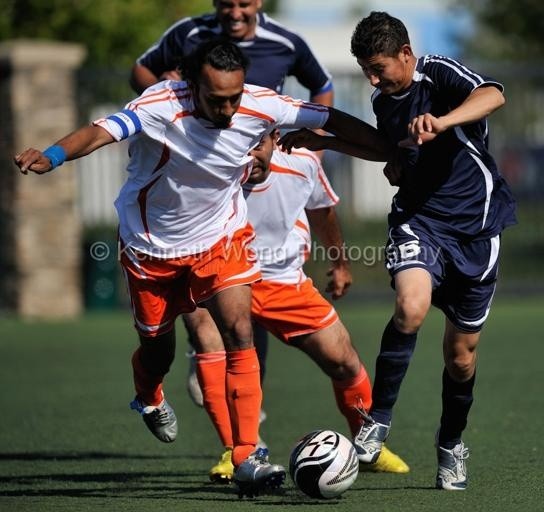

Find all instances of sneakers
(436, 439), (469, 491)
(129, 394), (178, 443)
(353, 395), (410, 474)
(208, 447), (286, 489)
(187, 368), (204, 408)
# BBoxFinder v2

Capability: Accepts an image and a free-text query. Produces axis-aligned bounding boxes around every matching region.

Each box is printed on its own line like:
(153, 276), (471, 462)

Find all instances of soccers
(290, 429), (359, 499)
